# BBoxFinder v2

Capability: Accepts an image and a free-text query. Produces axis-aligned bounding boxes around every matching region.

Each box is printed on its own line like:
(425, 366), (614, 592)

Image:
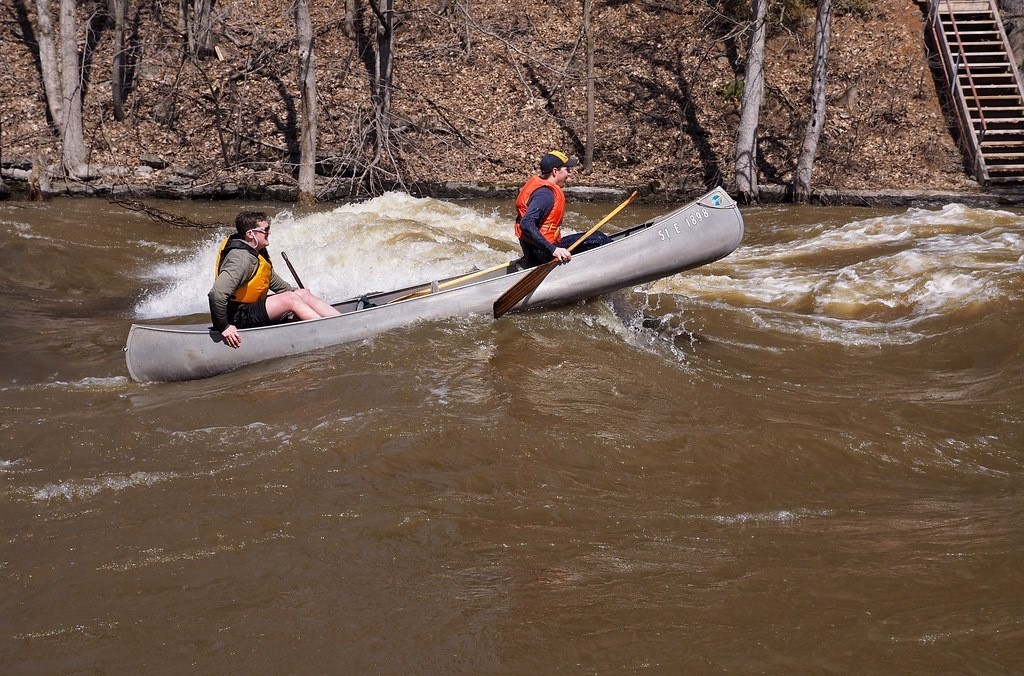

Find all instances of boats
(122, 185), (746, 383)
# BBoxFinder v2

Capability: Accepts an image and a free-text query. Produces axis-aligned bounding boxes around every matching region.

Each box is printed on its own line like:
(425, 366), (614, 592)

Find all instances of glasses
(248, 227), (270, 234)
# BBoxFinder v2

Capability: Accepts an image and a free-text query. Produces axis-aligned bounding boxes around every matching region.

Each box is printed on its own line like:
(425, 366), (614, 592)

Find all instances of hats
(540, 150), (579, 174)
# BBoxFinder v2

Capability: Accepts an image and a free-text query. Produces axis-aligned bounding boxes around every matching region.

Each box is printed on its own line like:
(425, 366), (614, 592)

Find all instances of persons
(208, 212), (340, 349)
(514, 150), (613, 265)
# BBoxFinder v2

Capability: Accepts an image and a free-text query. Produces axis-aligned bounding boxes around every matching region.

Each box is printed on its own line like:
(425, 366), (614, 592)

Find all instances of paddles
(387, 259), (519, 305)
(281, 251), (304, 289)
(493, 187), (640, 319)
